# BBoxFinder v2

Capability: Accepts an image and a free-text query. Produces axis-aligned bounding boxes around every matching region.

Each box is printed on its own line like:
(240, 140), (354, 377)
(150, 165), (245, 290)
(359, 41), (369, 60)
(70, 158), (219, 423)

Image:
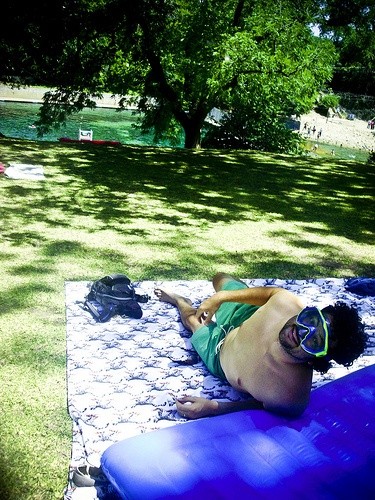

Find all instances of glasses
(296, 306), (328, 357)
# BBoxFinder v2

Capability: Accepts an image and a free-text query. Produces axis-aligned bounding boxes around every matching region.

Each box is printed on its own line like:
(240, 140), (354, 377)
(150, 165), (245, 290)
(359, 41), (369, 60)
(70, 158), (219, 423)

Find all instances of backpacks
(76, 275), (151, 323)
(344, 277), (375, 297)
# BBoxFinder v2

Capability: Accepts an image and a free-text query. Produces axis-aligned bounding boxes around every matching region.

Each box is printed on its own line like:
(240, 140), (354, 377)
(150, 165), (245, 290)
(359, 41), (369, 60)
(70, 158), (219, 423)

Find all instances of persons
(302, 121), (323, 139)
(155, 272), (365, 417)
